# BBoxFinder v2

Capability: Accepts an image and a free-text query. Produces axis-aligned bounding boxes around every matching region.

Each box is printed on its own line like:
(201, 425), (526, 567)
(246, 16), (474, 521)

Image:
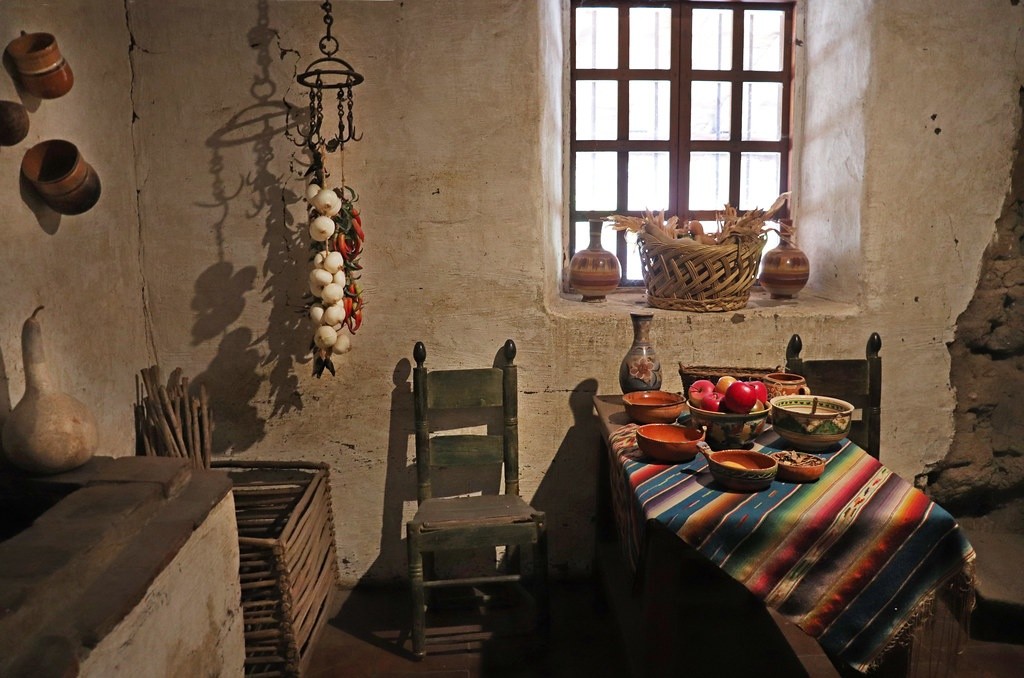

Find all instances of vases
(618, 306), (664, 396)
(569, 212), (621, 303)
(760, 220), (810, 300)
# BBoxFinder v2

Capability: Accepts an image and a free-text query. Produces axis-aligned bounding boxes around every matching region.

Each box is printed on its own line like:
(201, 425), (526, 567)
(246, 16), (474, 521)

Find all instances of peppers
(331, 208), (364, 335)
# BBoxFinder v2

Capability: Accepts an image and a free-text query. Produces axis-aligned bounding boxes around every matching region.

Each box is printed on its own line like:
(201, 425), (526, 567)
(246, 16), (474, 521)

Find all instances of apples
(750, 399), (764, 412)
(701, 392), (725, 412)
(744, 382), (767, 403)
(716, 376), (737, 394)
(688, 380), (715, 408)
(725, 382), (757, 412)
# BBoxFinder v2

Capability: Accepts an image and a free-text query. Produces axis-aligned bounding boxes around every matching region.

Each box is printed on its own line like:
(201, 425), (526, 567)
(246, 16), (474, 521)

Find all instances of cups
(763, 373), (810, 423)
(8, 31), (74, 99)
(22, 140), (101, 215)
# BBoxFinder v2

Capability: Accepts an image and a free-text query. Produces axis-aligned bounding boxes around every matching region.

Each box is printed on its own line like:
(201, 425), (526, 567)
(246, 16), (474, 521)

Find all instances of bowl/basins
(770, 395), (855, 451)
(708, 449), (778, 491)
(622, 390), (686, 423)
(636, 424), (707, 461)
(686, 398), (772, 448)
(770, 452), (824, 481)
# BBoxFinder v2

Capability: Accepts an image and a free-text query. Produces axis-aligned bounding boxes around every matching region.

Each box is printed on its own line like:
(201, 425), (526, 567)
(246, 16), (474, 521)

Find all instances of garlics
(306, 184), (352, 354)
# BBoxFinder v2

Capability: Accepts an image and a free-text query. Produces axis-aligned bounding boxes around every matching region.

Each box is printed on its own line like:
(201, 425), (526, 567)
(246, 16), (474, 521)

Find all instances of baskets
(637, 232), (767, 312)
(679, 361), (790, 400)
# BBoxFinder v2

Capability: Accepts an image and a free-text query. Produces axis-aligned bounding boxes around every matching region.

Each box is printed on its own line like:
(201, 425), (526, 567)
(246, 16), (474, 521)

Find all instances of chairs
(407, 339), (543, 662)
(786, 332), (883, 464)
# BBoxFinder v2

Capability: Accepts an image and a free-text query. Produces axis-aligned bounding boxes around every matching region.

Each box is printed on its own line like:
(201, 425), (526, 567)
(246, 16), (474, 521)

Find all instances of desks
(591, 394), (976, 678)
